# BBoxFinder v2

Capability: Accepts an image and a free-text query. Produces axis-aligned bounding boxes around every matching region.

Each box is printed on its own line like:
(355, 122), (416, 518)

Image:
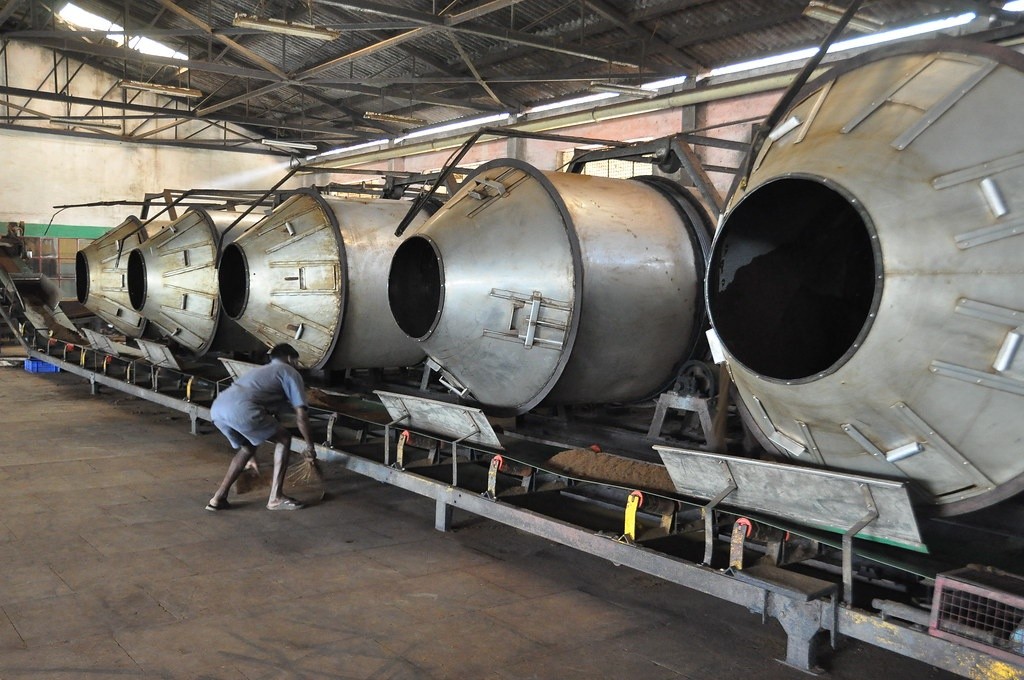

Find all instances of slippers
(206, 501), (231, 512)
(266, 498), (304, 511)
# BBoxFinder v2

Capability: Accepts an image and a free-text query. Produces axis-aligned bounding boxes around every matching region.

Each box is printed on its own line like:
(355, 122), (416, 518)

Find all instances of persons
(204, 342), (317, 511)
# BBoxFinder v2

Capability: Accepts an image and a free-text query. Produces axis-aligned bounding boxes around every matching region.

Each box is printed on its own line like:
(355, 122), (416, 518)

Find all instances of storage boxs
(24, 360), (60, 373)
(928, 563), (1024, 666)
